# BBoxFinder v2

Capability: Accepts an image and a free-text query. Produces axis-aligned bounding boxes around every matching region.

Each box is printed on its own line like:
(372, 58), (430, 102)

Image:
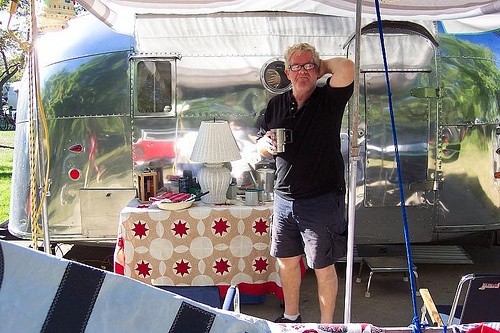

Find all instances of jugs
(247, 164), (275, 202)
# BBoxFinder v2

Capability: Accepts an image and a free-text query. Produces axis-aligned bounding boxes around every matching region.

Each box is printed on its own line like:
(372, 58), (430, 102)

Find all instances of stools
(356, 256), (421, 299)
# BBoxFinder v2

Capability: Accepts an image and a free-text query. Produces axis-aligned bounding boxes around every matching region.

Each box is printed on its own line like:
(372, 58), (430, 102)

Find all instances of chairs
(419, 273), (500, 328)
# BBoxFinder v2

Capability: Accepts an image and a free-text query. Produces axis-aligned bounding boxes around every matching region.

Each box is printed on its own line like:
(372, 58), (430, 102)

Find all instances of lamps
(190, 117), (241, 203)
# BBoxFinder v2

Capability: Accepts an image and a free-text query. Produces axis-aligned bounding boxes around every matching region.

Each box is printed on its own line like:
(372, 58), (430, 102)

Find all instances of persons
(256, 43), (356, 324)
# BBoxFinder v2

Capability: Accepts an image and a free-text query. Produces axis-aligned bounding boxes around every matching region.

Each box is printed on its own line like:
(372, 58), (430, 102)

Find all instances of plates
(156, 195), (196, 210)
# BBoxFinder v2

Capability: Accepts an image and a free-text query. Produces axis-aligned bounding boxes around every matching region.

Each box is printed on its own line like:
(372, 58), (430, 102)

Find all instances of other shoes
(273, 311), (302, 324)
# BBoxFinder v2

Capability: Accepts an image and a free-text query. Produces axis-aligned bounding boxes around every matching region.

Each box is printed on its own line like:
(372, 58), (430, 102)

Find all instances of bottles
(492, 127), (500, 182)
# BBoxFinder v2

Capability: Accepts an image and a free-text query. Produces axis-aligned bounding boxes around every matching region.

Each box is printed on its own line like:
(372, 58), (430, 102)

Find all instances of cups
(270, 128), (293, 153)
(226, 182), (238, 199)
(178, 178), (188, 193)
(244, 190), (258, 206)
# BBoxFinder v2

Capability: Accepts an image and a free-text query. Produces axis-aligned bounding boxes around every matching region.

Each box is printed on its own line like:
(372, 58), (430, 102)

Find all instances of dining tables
(113, 182), (307, 307)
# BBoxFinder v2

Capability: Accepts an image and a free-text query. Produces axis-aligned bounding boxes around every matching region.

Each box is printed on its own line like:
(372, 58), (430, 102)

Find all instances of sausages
(169, 193), (190, 202)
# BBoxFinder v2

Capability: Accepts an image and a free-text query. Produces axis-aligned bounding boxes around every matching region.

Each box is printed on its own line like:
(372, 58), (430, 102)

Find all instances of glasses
(288, 63), (318, 72)
